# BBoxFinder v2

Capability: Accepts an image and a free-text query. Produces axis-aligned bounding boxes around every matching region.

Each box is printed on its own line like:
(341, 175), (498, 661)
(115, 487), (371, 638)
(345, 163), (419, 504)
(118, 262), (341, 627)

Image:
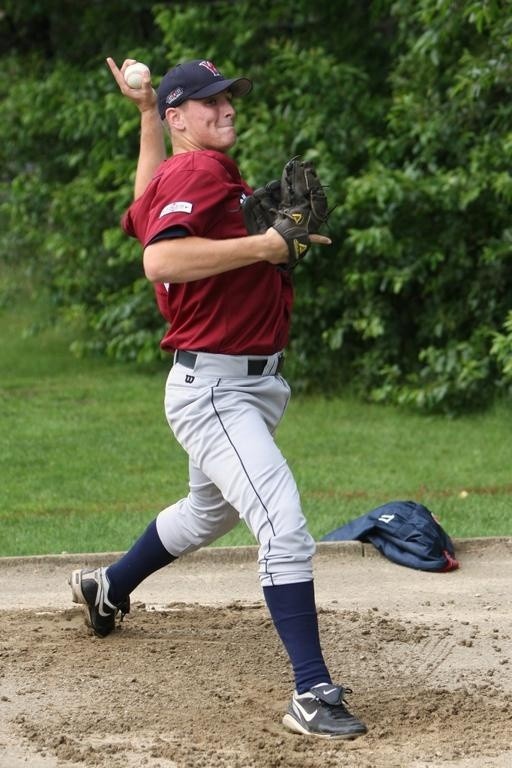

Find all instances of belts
(175, 350), (286, 376)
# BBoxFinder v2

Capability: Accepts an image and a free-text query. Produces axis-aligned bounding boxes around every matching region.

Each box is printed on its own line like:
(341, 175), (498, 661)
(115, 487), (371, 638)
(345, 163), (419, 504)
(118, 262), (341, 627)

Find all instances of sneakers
(68, 565), (130, 638)
(282, 682), (367, 741)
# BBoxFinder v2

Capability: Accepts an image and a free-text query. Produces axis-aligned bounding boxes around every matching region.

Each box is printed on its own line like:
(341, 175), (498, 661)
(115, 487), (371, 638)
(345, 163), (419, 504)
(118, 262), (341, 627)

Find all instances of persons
(67, 56), (369, 745)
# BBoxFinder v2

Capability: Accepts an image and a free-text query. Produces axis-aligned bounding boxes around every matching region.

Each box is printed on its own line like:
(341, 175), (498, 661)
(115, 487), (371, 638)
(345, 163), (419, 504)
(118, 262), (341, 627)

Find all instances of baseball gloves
(242, 159), (328, 275)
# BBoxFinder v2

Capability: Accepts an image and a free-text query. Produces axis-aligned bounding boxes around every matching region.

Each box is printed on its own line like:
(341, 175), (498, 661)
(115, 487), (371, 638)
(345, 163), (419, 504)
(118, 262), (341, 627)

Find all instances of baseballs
(125, 62), (150, 89)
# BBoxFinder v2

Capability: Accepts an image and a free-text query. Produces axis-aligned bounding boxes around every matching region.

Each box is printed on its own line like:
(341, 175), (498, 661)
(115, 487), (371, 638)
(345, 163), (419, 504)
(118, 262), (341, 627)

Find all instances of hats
(156, 60), (253, 121)
